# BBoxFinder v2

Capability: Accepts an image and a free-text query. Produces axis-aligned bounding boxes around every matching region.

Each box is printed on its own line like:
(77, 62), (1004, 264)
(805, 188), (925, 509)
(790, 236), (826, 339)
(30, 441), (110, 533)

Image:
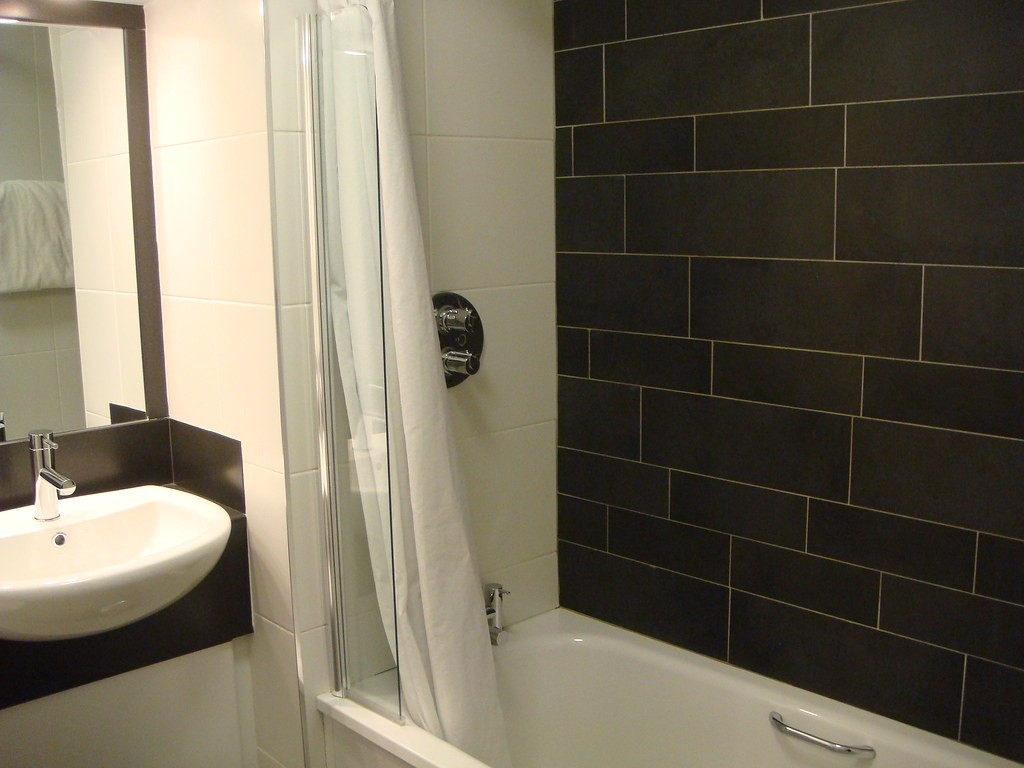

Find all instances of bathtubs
(314, 605), (1024, 768)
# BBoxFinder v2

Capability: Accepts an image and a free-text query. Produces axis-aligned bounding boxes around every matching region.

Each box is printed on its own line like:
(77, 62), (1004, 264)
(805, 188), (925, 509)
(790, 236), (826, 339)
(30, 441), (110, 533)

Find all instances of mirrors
(0, 1), (169, 443)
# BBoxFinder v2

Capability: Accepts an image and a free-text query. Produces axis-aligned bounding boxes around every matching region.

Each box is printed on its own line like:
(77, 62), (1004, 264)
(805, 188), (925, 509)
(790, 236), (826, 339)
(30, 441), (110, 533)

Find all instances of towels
(0, 179), (76, 294)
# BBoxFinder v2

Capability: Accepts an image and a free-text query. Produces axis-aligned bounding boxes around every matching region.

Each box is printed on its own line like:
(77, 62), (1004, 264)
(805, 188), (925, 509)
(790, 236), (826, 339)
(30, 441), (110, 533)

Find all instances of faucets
(0, 411), (6, 442)
(28, 429), (76, 521)
(488, 623), (509, 648)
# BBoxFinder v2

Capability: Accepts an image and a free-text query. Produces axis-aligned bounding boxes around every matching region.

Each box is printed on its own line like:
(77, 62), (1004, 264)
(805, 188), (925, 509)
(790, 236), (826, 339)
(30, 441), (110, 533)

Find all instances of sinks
(0, 485), (232, 639)
